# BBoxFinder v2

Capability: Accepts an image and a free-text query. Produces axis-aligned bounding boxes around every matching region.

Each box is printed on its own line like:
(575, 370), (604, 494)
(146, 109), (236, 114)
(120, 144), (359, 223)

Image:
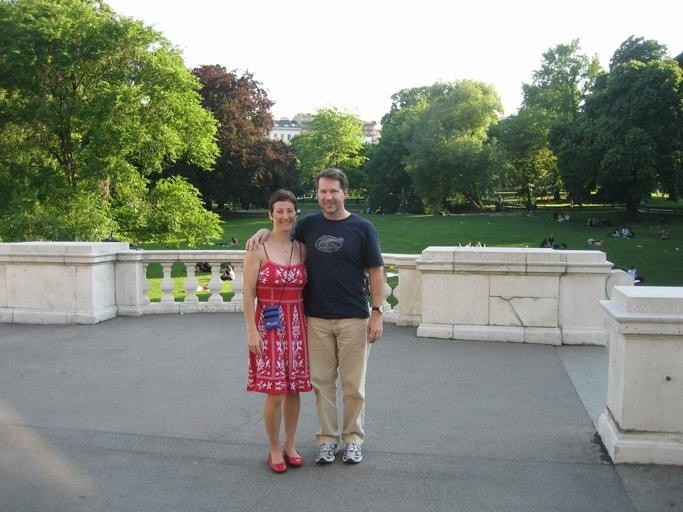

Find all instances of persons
(229, 237), (239, 247)
(220, 265), (235, 280)
(498, 194), (504, 204)
(456, 240), (487, 247)
(627, 266), (645, 282)
(540, 212), (672, 249)
(571, 200), (574, 207)
(196, 262), (212, 272)
(225, 206), (232, 214)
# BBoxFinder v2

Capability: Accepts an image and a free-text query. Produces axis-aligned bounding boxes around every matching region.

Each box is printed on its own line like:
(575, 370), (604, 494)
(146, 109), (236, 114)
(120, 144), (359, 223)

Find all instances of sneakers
(314, 444), (339, 464)
(341, 443), (363, 464)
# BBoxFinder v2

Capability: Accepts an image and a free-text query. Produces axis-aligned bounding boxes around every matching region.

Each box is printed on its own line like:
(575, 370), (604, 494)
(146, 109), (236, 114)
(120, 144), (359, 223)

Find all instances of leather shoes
(284, 448), (304, 466)
(268, 452), (287, 473)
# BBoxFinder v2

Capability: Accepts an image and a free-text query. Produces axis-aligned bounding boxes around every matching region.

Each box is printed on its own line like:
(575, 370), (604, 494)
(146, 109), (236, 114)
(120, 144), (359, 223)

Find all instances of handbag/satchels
(263, 307), (282, 330)
